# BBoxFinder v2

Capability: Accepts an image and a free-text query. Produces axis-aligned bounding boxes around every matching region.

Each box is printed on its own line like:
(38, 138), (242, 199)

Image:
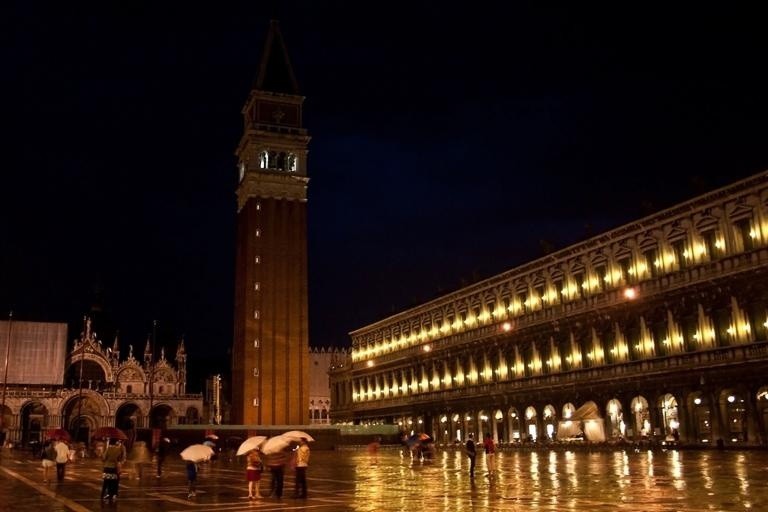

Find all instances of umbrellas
(207, 434), (219, 438)
(94, 427), (127, 438)
(235, 430), (316, 455)
(48, 426), (71, 441)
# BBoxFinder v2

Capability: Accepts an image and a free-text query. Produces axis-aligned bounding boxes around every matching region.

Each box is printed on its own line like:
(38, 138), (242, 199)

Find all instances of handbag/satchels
(465, 449), (475, 457)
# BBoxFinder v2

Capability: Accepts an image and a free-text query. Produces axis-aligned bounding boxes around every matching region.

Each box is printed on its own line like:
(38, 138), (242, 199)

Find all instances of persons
(246, 445), (264, 499)
(293, 437), (310, 499)
(469, 477), (479, 511)
(465, 434), (477, 477)
(482, 433), (497, 478)
(67, 441), (86, 464)
(263, 449), (291, 500)
(100, 438), (123, 504)
(368, 439), (381, 465)
(185, 461), (201, 498)
(53, 438), (70, 483)
(3, 439), (13, 455)
(211, 447), (218, 464)
(39, 441), (56, 482)
(488, 478), (497, 511)
(399, 429), (435, 465)
(154, 438), (170, 478)
(95, 441), (148, 481)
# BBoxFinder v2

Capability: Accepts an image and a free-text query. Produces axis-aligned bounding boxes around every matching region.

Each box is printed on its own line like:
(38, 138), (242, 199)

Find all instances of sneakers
(292, 493), (307, 498)
(188, 491), (196, 498)
(105, 494), (117, 500)
(249, 495), (264, 499)
